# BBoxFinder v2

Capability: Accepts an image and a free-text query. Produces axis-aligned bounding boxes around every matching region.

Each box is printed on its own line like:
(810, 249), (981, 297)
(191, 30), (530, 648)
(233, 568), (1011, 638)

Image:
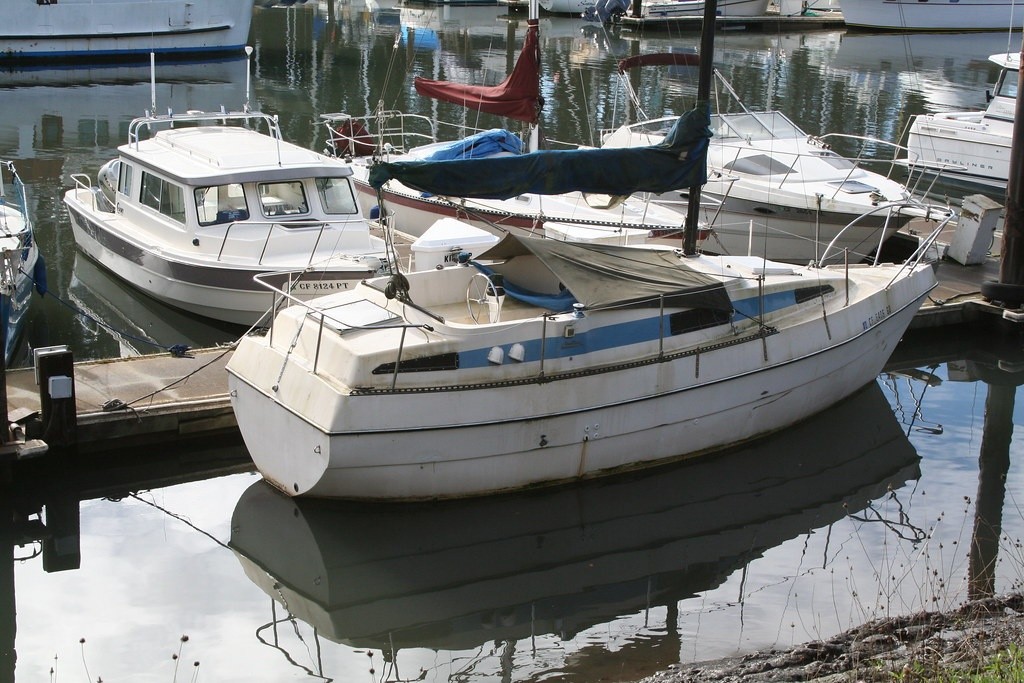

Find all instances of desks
(261, 196), (288, 208)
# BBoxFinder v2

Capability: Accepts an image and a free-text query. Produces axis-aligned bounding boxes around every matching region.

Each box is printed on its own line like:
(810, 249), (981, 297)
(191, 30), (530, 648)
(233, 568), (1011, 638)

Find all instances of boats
(227, 380), (924, 650)
(1, 161), (47, 368)
(63, 51), (401, 329)
(223, 1), (1024, 506)
(2, 0), (255, 54)
